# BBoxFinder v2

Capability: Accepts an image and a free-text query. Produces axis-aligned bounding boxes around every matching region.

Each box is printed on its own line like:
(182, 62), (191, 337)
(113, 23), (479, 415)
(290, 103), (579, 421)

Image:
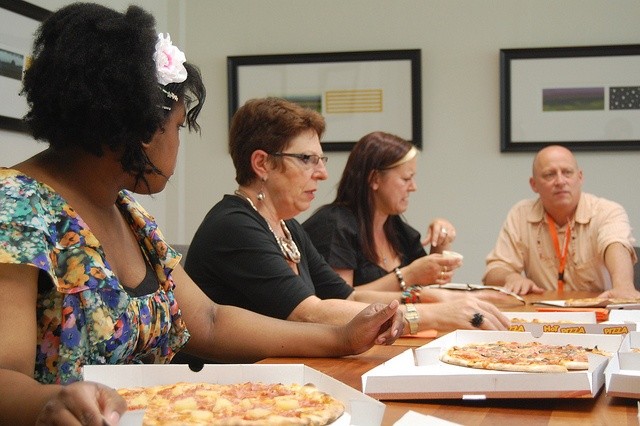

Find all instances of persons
(0, 2), (402, 426)
(301, 131), (463, 290)
(184, 96), (510, 330)
(484, 145), (640, 304)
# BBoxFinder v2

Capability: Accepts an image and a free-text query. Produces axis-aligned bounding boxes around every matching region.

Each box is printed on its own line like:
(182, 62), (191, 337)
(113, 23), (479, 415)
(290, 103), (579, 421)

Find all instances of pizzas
(442, 342), (610, 371)
(565, 298), (640, 303)
(511, 318), (563, 324)
(116, 382), (343, 426)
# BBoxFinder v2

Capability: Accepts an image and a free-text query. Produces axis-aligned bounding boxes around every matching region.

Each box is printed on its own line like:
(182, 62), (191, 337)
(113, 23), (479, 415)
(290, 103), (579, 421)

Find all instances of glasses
(265, 151), (328, 167)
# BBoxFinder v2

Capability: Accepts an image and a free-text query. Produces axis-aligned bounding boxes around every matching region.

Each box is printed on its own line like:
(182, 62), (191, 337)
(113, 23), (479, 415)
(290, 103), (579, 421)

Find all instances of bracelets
(395, 267), (407, 291)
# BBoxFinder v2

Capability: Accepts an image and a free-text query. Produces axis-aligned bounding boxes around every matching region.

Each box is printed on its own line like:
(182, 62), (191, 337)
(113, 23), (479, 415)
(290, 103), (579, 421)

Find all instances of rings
(441, 227), (448, 238)
(441, 272), (446, 279)
(443, 265), (448, 272)
(471, 313), (483, 328)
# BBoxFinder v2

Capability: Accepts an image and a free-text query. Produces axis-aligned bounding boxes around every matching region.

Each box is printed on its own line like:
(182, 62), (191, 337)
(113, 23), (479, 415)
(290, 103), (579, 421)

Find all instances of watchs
(404, 302), (420, 334)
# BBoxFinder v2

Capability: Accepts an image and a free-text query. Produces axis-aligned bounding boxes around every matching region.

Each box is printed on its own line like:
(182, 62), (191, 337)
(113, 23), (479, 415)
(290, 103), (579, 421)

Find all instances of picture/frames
(226, 49), (421, 152)
(499, 44), (640, 152)
(0, 0), (54, 133)
